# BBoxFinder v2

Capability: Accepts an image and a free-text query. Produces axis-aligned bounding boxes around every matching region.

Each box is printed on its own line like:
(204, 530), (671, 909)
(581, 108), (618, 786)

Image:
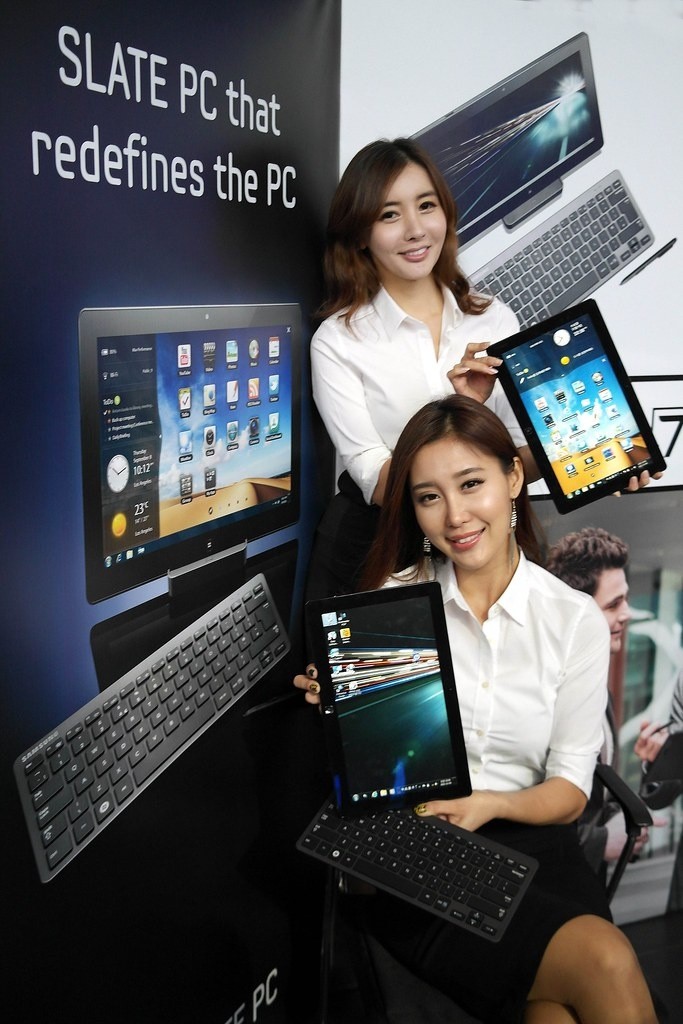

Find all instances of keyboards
(295, 782), (539, 945)
(462, 169), (656, 335)
(11, 571), (291, 884)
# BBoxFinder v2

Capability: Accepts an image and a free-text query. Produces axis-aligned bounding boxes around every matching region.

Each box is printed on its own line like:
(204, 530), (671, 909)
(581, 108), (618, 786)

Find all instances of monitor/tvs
(77, 302), (303, 696)
(394, 32), (605, 252)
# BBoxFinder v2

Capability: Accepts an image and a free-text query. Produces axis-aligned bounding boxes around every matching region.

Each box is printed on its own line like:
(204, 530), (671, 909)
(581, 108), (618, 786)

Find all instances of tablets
(485, 296), (669, 515)
(303, 579), (476, 819)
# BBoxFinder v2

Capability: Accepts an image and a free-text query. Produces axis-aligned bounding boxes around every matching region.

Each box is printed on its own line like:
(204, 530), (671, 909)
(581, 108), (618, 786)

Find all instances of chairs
(315, 765), (668, 1024)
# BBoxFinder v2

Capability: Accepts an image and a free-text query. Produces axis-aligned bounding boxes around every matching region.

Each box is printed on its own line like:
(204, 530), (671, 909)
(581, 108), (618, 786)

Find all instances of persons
(293, 394), (657, 1024)
(310, 138), (664, 595)
(543, 526), (667, 865)
(633, 669), (683, 811)
